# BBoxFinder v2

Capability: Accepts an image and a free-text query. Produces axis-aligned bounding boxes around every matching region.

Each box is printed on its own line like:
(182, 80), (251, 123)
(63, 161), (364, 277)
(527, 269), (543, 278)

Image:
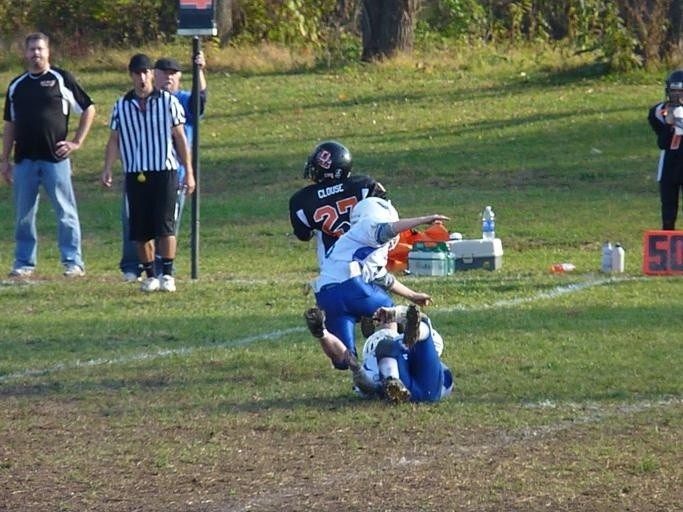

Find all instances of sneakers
(402, 303), (420, 346)
(361, 315), (375, 337)
(64, 267), (83, 277)
(383, 377), (411, 403)
(10, 269), (32, 277)
(304, 308), (326, 338)
(122, 272), (176, 292)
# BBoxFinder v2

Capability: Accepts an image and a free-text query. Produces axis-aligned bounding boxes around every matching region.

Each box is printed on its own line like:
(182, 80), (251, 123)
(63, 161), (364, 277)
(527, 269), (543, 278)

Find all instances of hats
(156, 57), (182, 71)
(129, 54), (150, 72)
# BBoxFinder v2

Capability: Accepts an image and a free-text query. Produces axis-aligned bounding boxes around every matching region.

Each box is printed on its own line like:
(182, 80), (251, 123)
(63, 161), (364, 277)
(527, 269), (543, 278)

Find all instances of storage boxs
(407, 251), (453, 277)
(447, 238), (504, 271)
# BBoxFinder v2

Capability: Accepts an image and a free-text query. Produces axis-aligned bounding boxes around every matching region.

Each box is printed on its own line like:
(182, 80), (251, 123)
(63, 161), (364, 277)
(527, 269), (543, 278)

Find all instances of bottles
(551, 263), (576, 273)
(408, 243), (456, 276)
(482, 205), (495, 239)
(611, 242), (625, 273)
(600, 239), (613, 273)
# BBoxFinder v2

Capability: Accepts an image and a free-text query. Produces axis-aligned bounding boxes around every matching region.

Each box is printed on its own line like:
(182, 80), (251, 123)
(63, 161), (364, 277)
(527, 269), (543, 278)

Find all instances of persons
(288, 142), (455, 404)
(118, 50), (207, 282)
(101, 53), (196, 292)
(0, 33), (96, 277)
(647, 70), (683, 230)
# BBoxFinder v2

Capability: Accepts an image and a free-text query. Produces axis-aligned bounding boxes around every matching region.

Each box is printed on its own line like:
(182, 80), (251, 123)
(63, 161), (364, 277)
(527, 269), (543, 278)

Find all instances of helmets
(351, 196), (401, 251)
(665, 70), (683, 105)
(304, 142), (352, 183)
(362, 328), (398, 361)
